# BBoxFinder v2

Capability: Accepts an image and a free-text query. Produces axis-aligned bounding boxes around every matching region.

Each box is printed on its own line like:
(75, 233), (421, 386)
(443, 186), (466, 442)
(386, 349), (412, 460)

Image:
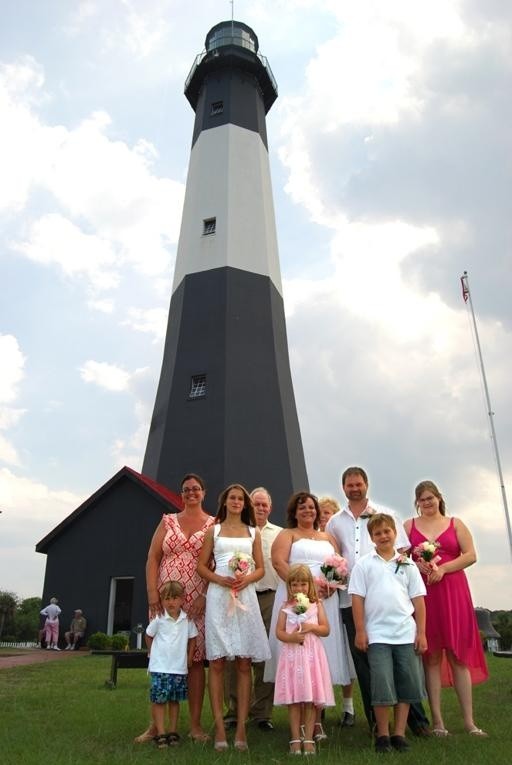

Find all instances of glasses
(180, 487), (204, 494)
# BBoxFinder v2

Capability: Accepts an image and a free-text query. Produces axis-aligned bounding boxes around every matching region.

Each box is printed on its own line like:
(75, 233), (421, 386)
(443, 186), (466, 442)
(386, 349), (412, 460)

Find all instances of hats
(74, 609), (83, 614)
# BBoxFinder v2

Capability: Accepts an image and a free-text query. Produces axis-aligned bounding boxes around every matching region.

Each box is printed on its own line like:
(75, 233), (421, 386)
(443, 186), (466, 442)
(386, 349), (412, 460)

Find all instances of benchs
(87, 646), (210, 690)
(38, 624), (88, 652)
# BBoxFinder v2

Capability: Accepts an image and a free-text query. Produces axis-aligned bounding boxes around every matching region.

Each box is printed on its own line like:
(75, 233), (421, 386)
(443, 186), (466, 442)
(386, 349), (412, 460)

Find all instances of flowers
(293, 591), (309, 647)
(226, 553), (255, 599)
(319, 553), (349, 600)
(413, 540), (440, 585)
(394, 552), (414, 574)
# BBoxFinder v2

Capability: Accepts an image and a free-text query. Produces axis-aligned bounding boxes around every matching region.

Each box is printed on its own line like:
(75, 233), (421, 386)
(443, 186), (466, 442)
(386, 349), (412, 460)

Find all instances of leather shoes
(218, 715), (274, 731)
(411, 728), (433, 737)
(344, 708), (356, 726)
(321, 708), (325, 722)
(371, 722), (409, 753)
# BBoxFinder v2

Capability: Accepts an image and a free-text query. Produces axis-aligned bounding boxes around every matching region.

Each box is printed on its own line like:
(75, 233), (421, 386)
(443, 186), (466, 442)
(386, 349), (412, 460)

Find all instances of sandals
(233, 734), (249, 754)
(188, 730), (211, 744)
(433, 726), (452, 736)
(134, 725), (180, 750)
(466, 727), (487, 736)
(289, 722), (328, 756)
(215, 732), (229, 753)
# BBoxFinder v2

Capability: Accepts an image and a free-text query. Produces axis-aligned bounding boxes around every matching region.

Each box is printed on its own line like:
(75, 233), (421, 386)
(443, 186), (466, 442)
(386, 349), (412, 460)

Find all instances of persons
(222, 486), (283, 732)
(144, 580), (199, 748)
(135, 472), (219, 745)
(276, 564), (336, 755)
(197, 484), (272, 753)
(263, 491), (357, 741)
(348, 513), (429, 754)
(317, 495), (358, 727)
(40, 598), (62, 651)
(403, 479), (490, 737)
(64, 609), (87, 651)
(35, 630), (44, 648)
(325, 466), (434, 740)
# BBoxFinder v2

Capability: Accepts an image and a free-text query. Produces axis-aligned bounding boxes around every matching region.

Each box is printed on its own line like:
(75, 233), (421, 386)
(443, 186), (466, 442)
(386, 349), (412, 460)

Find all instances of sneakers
(36, 642), (41, 649)
(65, 644), (75, 651)
(46, 645), (61, 651)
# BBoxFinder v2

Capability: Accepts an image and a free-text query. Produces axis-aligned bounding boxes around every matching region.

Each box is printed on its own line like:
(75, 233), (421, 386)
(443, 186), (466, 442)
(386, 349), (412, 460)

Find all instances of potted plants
(85, 630), (131, 658)
